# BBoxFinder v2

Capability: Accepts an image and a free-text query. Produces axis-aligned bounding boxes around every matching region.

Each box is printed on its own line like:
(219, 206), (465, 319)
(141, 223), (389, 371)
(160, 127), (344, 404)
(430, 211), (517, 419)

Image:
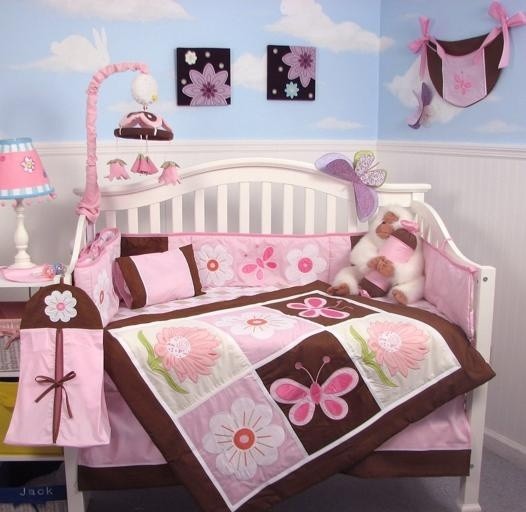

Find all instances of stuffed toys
(327, 203), (429, 304)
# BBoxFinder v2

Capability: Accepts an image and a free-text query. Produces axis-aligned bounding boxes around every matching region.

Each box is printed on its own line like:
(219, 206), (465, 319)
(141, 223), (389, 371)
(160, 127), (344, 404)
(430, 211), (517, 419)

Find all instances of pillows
(108, 234), (202, 313)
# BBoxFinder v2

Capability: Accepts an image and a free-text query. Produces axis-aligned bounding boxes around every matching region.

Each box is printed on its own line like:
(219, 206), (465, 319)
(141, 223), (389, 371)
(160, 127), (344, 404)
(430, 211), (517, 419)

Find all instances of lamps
(1, 137), (58, 275)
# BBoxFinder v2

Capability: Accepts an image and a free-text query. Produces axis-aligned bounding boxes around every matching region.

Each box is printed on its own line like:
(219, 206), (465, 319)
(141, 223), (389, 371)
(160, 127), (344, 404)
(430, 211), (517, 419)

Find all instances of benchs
(56, 160), (501, 511)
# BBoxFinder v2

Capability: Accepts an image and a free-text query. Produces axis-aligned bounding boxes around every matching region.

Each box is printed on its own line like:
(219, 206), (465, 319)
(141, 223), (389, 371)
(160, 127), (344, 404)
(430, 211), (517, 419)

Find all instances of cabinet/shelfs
(0, 270), (67, 503)
